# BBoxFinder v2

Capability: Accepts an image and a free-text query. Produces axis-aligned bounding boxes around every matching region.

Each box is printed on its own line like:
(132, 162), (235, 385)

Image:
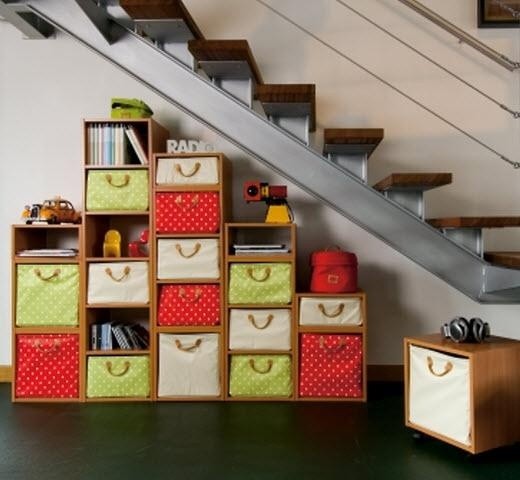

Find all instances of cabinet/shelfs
(403, 333), (520, 464)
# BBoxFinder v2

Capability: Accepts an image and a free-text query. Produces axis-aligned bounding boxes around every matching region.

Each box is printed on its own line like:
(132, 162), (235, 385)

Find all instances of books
(233, 243), (287, 249)
(86, 124), (148, 165)
(89, 322), (149, 350)
(17, 249), (77, 253)
(16, 252), (76, 257)
(234, 247), (291, 253)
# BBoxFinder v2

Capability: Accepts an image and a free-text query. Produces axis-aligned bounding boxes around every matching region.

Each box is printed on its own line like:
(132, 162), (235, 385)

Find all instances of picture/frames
(478, 0), (520, 28)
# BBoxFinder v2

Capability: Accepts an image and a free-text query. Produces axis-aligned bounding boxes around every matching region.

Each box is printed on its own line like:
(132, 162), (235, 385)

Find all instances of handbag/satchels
(110, 97), (153, 118)
(310, 244), (358, 293)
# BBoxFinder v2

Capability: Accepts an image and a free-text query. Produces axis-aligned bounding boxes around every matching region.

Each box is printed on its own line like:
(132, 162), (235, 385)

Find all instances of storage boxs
(409, 346), (472, 448)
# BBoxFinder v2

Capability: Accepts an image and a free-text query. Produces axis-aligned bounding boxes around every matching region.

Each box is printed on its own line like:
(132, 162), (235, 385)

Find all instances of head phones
(444, 318), (490, 344)
